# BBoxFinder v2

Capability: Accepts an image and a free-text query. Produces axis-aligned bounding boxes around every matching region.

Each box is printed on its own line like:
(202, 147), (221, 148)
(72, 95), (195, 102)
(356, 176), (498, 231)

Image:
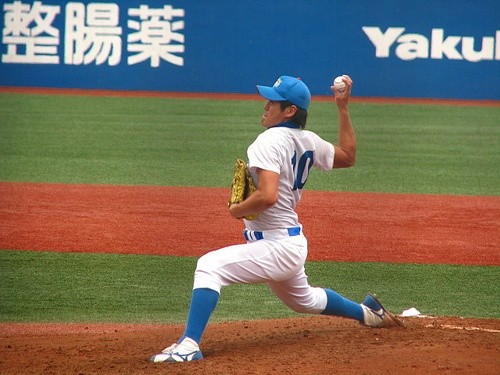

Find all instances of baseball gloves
(229, 159), (261, 221)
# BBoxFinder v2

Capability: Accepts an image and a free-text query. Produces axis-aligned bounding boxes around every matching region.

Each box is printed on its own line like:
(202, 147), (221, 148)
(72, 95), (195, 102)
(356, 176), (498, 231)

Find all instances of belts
(244, 226), (300, 241)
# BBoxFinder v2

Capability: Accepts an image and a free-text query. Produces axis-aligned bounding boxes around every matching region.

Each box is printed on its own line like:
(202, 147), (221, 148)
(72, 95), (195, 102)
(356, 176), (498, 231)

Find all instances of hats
(256, 77), (311, 110)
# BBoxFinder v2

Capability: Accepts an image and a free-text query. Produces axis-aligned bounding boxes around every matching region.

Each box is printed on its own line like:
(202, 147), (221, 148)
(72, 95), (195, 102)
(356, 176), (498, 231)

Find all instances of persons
(150, 74), (407, 364)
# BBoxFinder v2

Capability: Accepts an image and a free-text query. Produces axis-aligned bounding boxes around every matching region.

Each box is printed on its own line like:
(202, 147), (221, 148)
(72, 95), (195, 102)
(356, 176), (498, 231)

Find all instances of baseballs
(333, 75), (348, 91)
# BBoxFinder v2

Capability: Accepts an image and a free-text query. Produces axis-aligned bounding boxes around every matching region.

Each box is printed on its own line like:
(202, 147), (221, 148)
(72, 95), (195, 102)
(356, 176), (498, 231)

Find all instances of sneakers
(361, 291), (406, 327)
(149, 336), (204, 364)
